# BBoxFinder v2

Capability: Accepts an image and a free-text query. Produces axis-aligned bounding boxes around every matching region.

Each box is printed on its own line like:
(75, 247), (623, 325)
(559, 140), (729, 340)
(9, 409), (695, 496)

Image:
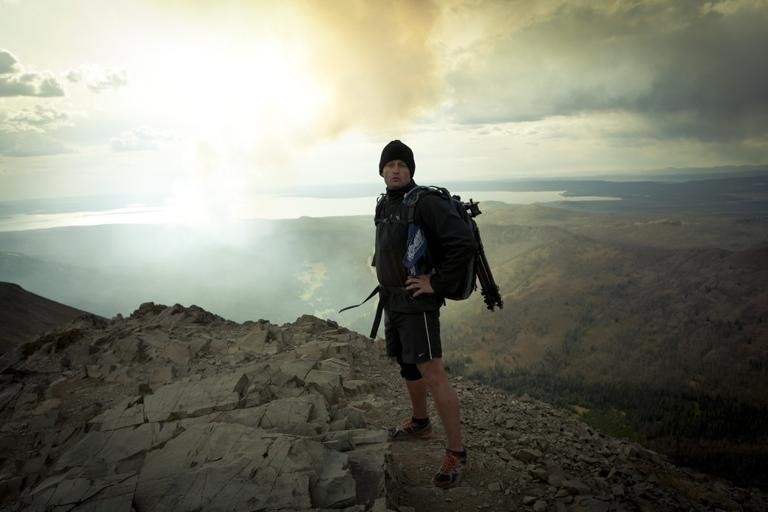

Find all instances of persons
(370, 139), (477, 489)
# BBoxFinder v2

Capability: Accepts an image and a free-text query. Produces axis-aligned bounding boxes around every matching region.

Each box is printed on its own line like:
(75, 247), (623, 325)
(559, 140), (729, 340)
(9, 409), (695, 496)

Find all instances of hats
(380, 140), (415, 179)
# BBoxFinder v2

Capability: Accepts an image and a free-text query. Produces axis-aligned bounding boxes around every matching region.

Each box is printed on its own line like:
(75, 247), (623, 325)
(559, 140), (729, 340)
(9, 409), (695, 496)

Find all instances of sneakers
(432, 445), (468, 487)
(388, 417), (432, 438)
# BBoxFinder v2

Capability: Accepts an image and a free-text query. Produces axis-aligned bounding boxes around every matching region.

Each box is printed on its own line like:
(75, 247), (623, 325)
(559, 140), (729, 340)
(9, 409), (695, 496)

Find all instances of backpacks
(403, 186), (476, 299)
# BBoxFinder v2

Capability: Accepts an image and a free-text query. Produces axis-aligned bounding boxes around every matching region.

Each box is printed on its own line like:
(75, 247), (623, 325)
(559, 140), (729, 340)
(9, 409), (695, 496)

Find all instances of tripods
(458, 199), (503, 313)
